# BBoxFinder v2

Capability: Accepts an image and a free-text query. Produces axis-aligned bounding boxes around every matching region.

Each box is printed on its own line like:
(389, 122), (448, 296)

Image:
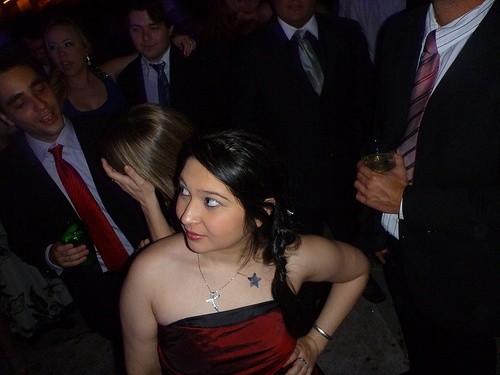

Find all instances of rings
(190, 41), (192, 43)
(60, 250), (67, 256)
(187, 41), (190, 43)
(298, 357), (306, 365)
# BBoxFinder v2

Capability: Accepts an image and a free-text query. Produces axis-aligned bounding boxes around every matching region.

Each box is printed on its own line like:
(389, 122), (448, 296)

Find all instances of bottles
(60, 219), (97, 270)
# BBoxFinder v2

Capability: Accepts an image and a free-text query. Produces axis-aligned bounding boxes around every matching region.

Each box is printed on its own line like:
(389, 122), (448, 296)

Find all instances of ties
(48, 143), (130, 273)
(293, 29), (325, 96)
(149, 61), (170, 108)
(393, 28), (441, 186)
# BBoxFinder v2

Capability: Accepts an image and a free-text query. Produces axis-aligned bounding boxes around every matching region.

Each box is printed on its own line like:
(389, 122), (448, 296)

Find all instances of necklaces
(198, 246), (254, 310)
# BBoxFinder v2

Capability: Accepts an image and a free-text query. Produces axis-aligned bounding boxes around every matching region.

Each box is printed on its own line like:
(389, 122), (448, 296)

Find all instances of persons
(0, 0), (500, 375)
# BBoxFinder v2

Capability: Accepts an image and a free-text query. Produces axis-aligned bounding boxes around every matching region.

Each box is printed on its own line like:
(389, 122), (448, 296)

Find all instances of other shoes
(362, 275), (385, 304)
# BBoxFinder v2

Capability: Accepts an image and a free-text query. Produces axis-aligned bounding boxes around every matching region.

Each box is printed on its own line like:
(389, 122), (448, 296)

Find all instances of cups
(362, 139), (395, 173)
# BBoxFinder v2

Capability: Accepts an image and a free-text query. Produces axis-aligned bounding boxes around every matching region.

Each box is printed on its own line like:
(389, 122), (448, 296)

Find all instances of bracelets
(313, 322), (333, 340)
